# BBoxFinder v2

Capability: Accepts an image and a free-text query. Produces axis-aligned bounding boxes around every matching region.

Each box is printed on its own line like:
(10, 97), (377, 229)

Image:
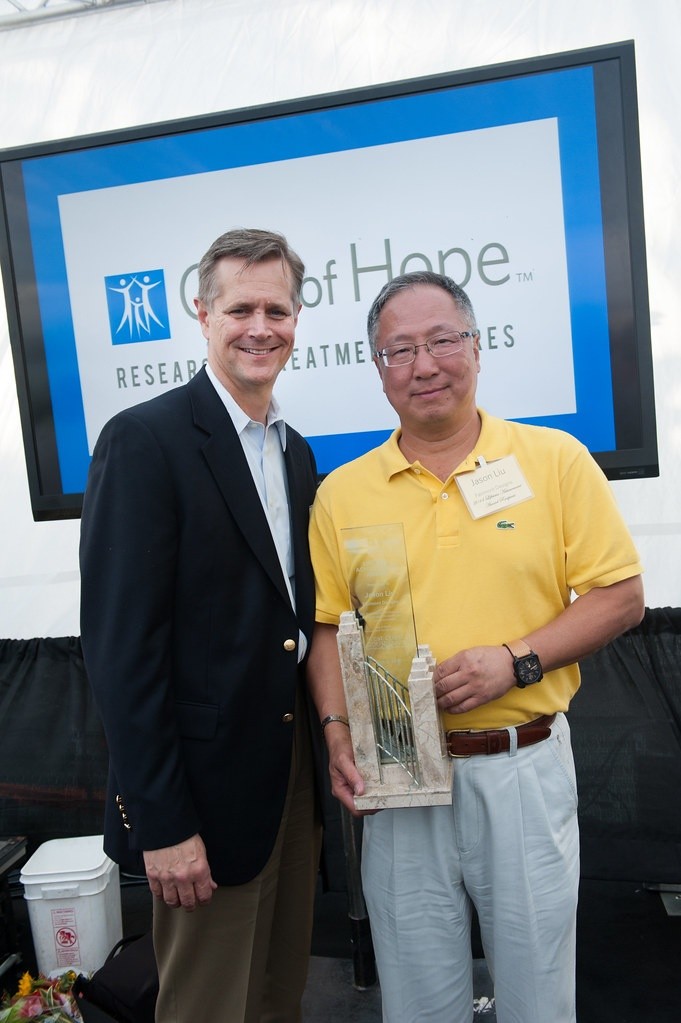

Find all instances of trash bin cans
(19, 835), (122, 980)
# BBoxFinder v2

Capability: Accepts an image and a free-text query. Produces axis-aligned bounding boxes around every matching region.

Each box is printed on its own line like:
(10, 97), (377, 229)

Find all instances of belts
(378, 713), (556, 758)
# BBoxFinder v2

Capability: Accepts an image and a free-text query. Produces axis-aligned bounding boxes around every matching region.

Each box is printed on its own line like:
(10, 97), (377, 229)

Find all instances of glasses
(375, 330), (473, 368)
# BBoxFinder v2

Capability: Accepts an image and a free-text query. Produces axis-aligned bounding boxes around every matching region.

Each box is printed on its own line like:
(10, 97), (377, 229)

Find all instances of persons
(79, 230), (325, 1023)
(308, 270), (642, 1023)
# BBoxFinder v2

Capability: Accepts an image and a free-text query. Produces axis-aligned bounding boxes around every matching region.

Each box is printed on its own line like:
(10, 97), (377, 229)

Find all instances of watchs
(502, 638), (543, 689)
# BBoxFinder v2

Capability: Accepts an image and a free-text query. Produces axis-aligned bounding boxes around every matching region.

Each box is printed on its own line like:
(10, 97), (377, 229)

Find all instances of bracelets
(321, 715), (349, 737)
